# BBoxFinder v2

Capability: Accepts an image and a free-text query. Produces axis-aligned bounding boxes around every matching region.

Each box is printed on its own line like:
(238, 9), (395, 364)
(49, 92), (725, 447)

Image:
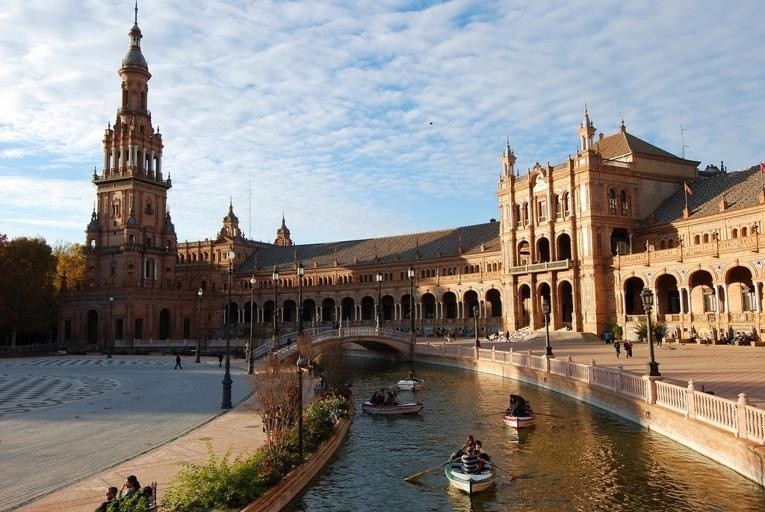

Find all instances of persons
(508, 394), (532, 417)
(174, 352), (183, 370)
(435, 327), (462, 341)
(369, 388), (400, 406)
(719, 328), (758, 346)
(506, 331), (511, 343)
(218, 352), (224, 367)
(271, 354), (279, 374)
(117, 475), (141, 503)
(613, 338), (633, 359)
(94, 487), (121, 512)
(456, 435), (486, 475)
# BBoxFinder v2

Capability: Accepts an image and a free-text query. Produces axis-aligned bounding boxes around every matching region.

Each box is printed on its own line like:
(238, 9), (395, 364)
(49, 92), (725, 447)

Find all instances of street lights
(272, 263), (279, 336)
(472, 306), (480, 346)
(640, 289), (661, 376)
(294, 353), (309, 462)
(246, 273), (256, 374)
(194, 287), (203, 363)
(375, 270), (383, 328)
(407, 266), (415, 332)
(221, 246), (235, 409)
(297, 261), (305, 335)
(542, 303), (553, 355)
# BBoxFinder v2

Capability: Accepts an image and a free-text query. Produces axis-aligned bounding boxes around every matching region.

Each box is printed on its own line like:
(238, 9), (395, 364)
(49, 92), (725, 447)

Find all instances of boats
(397, 379), (424, 391)
(445, 450), (495, 495)
(503, 414), (536, 430)
(362, 400), (423, 415)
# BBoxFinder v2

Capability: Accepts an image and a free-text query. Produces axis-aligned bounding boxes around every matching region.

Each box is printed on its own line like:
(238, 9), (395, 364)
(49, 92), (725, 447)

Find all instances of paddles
(404, 456), (462, 482)
(478, 458), (519, 478)
(527, 413), (565, 419)
(473, 412), (505, 421)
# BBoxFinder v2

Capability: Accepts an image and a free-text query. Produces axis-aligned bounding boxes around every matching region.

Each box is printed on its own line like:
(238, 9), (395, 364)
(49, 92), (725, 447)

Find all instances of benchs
(104, 481), (158, 512)
(313, 382), (323, 398)
